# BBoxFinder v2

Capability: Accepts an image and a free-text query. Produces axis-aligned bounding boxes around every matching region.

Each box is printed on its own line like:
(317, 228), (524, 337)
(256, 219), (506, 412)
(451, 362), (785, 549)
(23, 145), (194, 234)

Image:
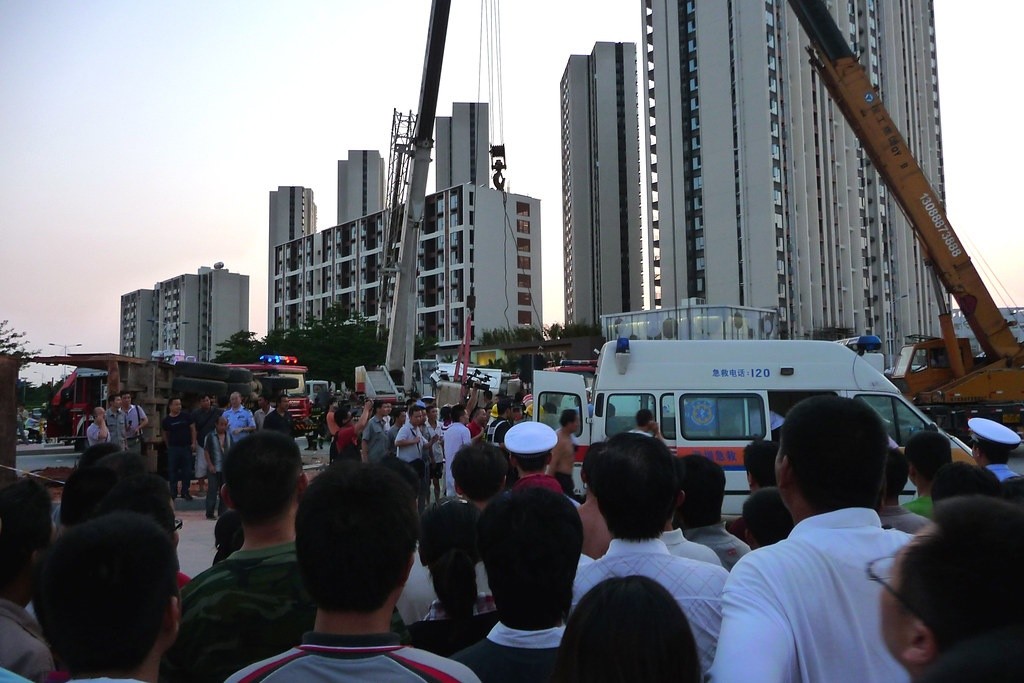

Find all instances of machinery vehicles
(331, 0), (458, 448)
(781, 0), (1024, 451)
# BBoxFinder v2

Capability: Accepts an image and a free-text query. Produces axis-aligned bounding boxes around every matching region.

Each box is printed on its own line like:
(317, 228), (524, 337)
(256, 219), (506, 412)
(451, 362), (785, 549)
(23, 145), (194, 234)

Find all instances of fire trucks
(41, 349), (331, 450)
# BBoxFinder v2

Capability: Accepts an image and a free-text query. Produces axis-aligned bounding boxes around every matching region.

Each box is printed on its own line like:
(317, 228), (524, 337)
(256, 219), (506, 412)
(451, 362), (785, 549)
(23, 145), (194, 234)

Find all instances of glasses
(174, 520), (182, 534)
(866, 553), (940, 642)
(421, 398), (433, 402)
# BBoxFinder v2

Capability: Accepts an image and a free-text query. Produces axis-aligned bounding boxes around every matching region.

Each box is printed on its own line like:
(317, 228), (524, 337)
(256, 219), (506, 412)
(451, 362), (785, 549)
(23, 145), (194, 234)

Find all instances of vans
(531, 329), (981, 536)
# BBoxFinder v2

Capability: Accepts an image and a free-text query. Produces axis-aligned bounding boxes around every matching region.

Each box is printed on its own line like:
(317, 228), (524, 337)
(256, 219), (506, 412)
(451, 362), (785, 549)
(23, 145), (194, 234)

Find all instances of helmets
(524, 404), (533, 417)
(491, 404), (498, 417)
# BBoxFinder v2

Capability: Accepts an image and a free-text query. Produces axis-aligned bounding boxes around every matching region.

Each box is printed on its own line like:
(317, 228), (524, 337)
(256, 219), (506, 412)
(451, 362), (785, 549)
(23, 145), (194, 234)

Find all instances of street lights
(48, 343), (83, 384)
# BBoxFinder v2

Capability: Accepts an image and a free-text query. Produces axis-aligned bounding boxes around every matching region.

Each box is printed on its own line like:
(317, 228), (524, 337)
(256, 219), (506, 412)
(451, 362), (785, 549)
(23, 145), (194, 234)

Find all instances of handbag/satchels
(409, 460), (426, 475)
(138, 437), (145, 452)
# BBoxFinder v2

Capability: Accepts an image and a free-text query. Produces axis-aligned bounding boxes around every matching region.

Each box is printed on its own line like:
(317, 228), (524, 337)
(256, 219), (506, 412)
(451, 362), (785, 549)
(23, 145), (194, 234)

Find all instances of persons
(705, 396), (916, 683)
(548, 392), (593, 501)
(304, 385), (557, 513)
(87, 390), (149, 453)
(0, 442), (190, 683)
(158, 431), (412, 683)
(409, 421), (793, 683)
(880, 418), (1024, 683)
(17, 404), (40, 444)
(33, 513), (181, 683)
(162, 392), (294, 519)
(224, 462), (480, 683)
(629, 409), (663, 442)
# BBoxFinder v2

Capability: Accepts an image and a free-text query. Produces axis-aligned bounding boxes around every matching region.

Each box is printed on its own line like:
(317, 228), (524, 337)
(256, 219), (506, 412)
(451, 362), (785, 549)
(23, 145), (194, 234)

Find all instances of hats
(968, 418), (1021, 450)
(503, 421), (559, 458)
(421, 396), (435, 399)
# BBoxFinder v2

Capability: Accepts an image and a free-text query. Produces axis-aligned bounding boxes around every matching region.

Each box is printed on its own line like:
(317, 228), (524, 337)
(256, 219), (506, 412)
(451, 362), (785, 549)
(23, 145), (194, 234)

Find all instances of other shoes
(181, 492), (192, 500)
(206, 512), (216, 520)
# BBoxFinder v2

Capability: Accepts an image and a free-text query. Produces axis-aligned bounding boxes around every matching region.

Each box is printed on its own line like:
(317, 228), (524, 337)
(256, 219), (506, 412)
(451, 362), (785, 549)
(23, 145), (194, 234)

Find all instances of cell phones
(175, 519), (182, 529)
